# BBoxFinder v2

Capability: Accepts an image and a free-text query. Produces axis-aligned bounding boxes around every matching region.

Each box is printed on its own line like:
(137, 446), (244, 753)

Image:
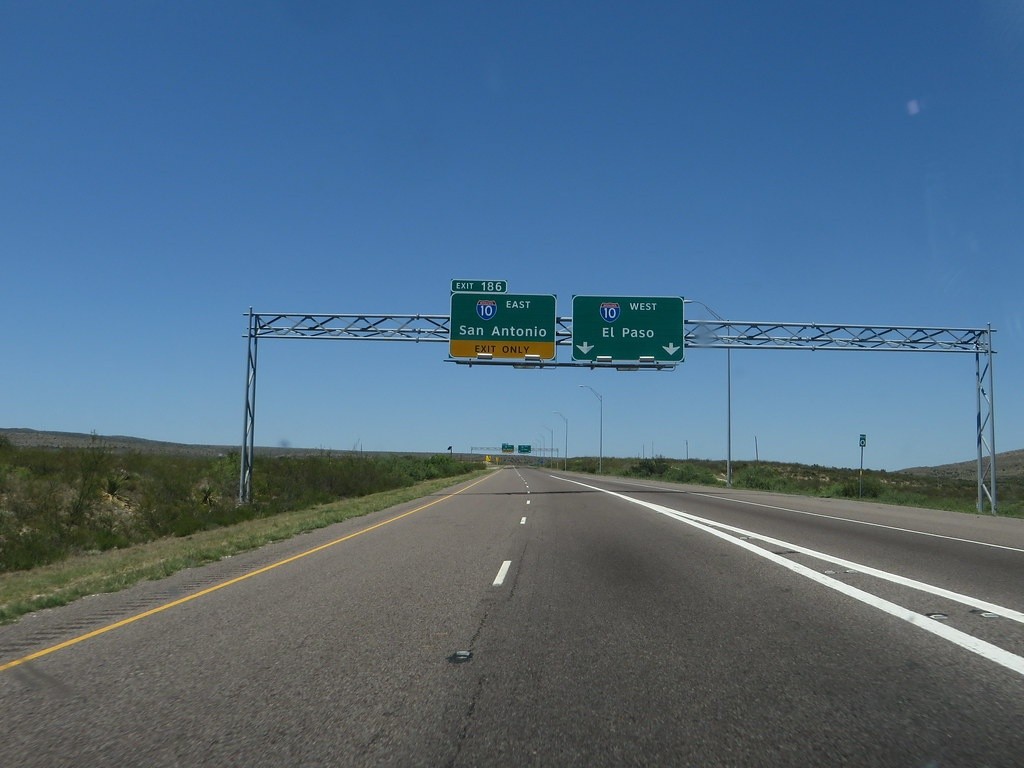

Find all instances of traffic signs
(572, 295), (684, 365)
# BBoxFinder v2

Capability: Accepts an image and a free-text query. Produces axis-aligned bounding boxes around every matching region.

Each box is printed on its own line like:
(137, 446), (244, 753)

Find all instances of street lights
(552, 411), (568, 471)
(685, 298), (732, 487)
(579, 384), (603, 475)
(531, 425), (553, 469)
